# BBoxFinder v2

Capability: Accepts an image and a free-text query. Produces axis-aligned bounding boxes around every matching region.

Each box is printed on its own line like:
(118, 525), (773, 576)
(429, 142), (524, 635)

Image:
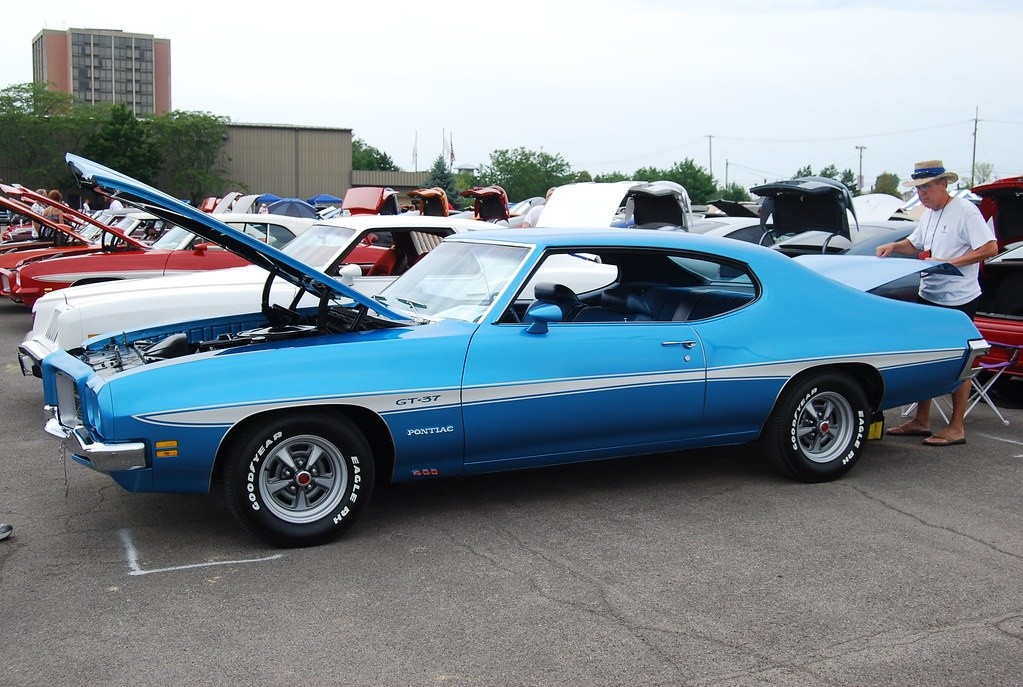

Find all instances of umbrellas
(267, 198), (315, 218)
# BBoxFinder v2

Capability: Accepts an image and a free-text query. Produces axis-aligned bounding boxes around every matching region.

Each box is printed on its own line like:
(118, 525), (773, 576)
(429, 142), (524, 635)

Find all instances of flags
(451, 142), (456, 163)
(412, 141), (417, 166)
(443, 135), (449, 154)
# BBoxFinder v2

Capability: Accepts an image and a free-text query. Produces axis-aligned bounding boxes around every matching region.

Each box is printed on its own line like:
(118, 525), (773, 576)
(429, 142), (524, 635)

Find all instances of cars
(0, 177), (401, 308)
(15, 179), (656, 380)
(354, 175), (1023, 387)
(40, 149), (991, 549)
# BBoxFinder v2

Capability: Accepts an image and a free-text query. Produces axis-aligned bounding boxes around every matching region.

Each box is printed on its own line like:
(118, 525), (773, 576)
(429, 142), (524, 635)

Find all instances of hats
(902, 160), (958, 187)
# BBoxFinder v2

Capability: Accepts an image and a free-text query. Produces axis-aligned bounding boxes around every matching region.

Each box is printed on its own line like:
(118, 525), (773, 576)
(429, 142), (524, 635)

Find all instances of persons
(520, 186), (556, 228)
(258, 203), (268, 215)
(231, 195), (241, 211)
(103, 194), (123, 210)
(83, 199), (91, 211)
(875, 160), (998, 447)
(30, 188), (48, 239)
(406, 205), (416, 213)
(44, 190), (65, 239)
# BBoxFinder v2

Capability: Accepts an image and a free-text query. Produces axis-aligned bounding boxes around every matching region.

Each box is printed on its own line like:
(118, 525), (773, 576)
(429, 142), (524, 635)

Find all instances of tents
(254, 192), (281, 202)
(306, 193), (343, 207)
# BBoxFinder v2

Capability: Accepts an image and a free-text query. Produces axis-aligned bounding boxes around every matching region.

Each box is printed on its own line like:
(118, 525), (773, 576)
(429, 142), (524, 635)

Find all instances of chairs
(573, 287), (652, 322)
(366, 248), (428, 276)
(521, 283), (587, 323)
(900, 327), (1023, 426)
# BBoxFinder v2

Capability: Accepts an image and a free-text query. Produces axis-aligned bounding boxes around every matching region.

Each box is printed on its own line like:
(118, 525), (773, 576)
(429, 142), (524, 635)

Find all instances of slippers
(922, 431), (965, 446)
(886, 425), (934, 437)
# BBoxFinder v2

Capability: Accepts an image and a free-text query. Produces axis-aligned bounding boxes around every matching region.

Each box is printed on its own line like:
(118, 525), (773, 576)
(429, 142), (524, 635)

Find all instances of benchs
(642, 286), (754, 322)
